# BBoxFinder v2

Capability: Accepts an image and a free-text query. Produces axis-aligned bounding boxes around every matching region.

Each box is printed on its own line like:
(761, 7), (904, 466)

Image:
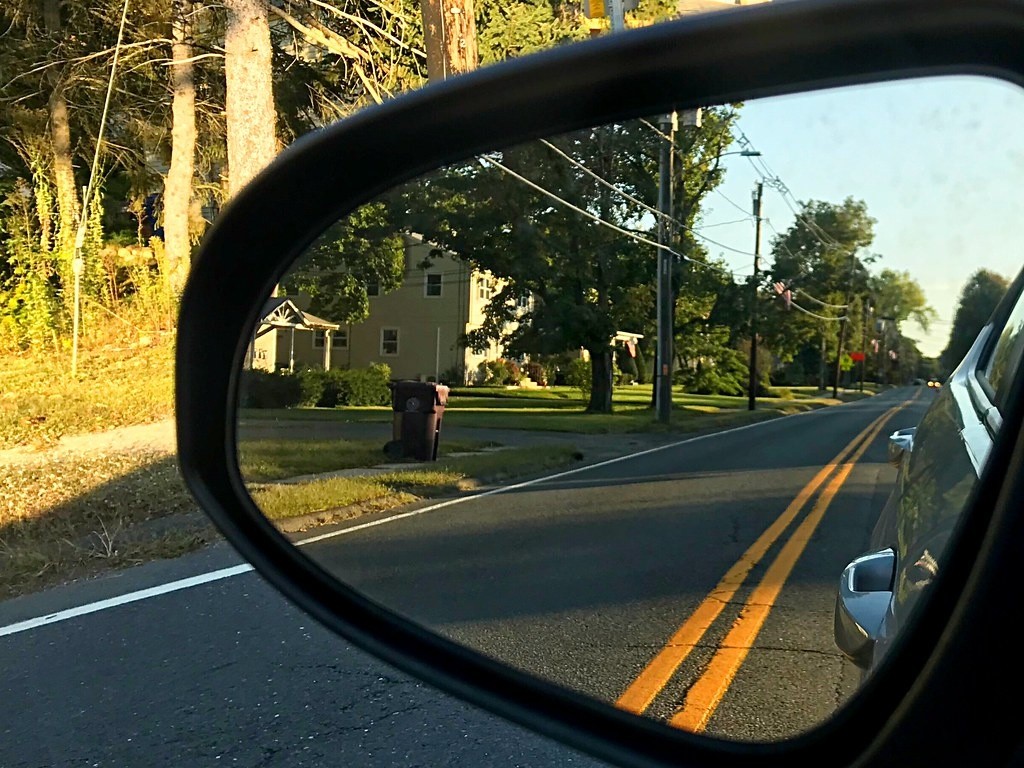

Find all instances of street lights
(655, 150), (761, 422)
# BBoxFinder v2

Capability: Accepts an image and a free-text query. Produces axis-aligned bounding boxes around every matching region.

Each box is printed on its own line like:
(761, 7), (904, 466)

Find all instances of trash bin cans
(389, 379), (449, 461)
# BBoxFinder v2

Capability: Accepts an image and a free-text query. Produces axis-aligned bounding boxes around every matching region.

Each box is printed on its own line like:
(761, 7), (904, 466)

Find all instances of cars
(930, 378), (939, 387)
(914, 379), (921, 385)
